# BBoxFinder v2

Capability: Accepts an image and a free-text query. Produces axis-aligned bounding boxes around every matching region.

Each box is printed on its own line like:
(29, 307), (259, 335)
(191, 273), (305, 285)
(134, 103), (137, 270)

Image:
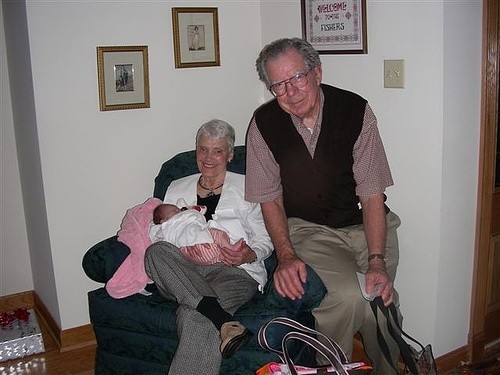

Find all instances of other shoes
(220, 320), (253, 359)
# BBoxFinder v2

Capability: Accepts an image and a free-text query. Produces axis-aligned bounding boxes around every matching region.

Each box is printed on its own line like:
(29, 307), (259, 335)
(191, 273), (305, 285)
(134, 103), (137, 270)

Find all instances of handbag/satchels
(370, 296), (438, 375)
(258, 317), (373, 375)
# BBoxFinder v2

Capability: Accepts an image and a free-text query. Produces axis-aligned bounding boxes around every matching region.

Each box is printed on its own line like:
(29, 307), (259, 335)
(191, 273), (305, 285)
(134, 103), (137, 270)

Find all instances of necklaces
(199, 176), (223, 197)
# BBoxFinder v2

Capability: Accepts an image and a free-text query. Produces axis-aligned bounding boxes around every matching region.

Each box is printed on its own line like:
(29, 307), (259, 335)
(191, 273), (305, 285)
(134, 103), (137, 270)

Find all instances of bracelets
(368, 254), (386, 264)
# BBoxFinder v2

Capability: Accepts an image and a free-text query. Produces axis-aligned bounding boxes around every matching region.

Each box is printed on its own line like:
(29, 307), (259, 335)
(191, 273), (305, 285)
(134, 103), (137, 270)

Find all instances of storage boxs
(0, 308), (45, 362)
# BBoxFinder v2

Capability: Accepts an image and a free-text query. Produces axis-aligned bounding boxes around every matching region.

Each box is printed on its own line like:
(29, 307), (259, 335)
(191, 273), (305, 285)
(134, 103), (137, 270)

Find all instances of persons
(153, 204), (244, 265)
(191, 26), (200, 51)
(118, 65), (128, 90)
(144, 119), (274, 375)
(244, 38), (403, 375)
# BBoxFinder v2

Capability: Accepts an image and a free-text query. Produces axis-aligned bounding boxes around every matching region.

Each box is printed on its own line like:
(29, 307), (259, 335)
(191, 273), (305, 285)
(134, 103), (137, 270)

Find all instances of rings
(232, 261), (235, 266)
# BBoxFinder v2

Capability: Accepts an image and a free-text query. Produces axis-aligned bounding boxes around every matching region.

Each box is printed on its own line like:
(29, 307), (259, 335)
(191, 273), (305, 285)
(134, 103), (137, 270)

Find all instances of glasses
(269, 67), (313, 97)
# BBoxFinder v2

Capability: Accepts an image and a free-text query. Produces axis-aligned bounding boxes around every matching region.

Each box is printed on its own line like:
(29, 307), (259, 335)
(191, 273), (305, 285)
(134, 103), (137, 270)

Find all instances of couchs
(82, 146), (327, 375)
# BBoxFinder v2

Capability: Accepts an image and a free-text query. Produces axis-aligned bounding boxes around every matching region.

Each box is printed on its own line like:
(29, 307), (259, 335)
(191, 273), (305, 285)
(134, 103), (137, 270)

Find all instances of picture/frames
(172, 7), (221, 69)
(96, 45), (150, 112)
(301, 0), (369, 54)
